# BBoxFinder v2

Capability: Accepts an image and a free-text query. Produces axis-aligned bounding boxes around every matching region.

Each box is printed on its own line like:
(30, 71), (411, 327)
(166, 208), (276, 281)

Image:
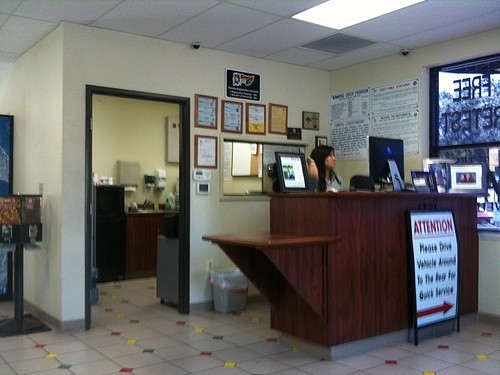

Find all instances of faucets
(143, 198), (151, 209)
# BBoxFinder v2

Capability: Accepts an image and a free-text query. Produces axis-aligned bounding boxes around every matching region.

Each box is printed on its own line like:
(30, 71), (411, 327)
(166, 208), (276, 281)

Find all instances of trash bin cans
(211, 267), (249, 314)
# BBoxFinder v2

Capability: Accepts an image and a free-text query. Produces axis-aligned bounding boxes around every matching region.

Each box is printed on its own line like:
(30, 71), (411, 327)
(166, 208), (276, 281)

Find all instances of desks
(202, 189), (490, 359)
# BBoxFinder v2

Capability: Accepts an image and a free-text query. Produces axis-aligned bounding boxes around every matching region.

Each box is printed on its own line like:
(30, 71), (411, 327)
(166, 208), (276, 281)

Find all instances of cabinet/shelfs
(125, 213), (178, 280)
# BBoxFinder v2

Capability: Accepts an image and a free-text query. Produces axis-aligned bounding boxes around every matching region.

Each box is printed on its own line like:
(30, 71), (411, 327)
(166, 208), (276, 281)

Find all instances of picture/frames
(315, 136), (327, 147)
(221, 100), (243, 133)
(268, 103), (288, 135)
(194, 135), (218, 169)
(450, 162), (487, 189)
(194, 93), (218, 129)
(246, 103), (266, 135)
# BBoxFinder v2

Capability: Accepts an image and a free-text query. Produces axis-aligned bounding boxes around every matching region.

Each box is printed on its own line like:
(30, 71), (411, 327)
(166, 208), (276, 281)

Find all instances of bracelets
(308, 160), (315, 164)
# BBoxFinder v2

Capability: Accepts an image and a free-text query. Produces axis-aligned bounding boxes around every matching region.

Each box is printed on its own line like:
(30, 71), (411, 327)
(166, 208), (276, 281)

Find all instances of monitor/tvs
(368, 136), (404, 192)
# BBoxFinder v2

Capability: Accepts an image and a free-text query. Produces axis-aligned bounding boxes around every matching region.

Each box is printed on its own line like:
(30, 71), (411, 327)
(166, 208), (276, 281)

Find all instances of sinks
(139, 210), (158, 212)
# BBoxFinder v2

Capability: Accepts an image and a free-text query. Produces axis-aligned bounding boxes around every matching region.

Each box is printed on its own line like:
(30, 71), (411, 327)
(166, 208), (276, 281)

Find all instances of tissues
(117, 160), (143, 193)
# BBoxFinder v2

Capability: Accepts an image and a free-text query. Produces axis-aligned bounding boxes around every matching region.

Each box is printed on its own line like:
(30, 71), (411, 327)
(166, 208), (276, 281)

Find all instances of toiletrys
(165, 192), (174, 210)
(154, 200), (159, 210)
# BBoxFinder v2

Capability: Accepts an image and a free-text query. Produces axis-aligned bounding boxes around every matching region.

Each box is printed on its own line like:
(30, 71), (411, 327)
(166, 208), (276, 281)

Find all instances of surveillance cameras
(402, 50), (408, 55)
(193, 43), (200, 48)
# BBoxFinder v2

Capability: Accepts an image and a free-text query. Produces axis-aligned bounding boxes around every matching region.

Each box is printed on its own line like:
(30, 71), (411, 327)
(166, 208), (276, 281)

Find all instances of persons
(460, 173), (473, 183)
(307, 146), (342, 191)
(287, 169), (295, 179)
